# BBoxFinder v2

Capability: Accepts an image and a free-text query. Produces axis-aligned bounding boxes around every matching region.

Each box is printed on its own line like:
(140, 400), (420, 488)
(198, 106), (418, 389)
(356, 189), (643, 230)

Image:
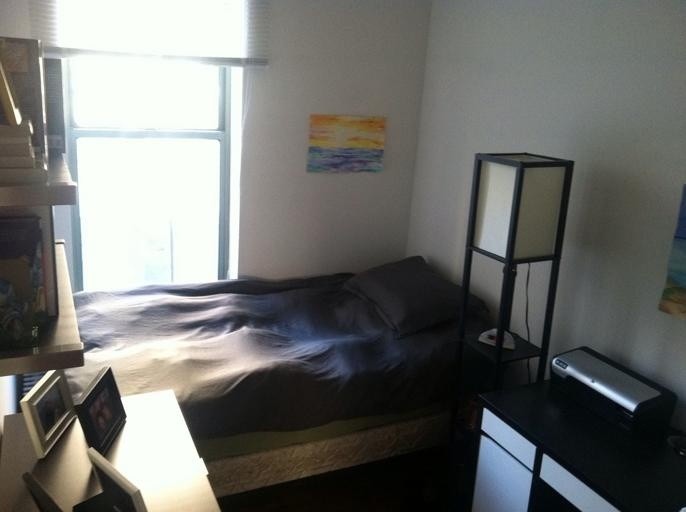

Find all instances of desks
(468, 377), (685, 511)
(1, 389), (224, 512)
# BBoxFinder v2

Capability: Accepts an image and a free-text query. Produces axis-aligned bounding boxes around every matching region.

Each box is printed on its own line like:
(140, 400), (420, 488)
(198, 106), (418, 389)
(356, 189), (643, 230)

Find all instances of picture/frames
(18, 364), (75, 459)
(73, 364), (128, 457)
(72, 446), (148, 512)
(21, 471), (64, 512)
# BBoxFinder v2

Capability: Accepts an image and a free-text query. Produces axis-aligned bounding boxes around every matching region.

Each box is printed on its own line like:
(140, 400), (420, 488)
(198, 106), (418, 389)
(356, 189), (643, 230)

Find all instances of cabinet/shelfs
(0, 147), (85, 378)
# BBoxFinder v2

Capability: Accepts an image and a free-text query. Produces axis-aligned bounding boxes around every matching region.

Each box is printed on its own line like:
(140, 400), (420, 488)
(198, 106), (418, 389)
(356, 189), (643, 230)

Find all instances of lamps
(441, 151), (579, 462)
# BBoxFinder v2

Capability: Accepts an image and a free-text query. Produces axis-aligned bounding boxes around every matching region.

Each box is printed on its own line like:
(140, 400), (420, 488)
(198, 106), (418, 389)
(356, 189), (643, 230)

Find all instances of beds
(17, 254), (503, 498)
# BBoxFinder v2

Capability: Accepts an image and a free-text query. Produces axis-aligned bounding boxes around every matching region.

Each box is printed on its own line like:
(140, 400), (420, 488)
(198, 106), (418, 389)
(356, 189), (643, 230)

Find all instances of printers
(551, 345), (668, 439)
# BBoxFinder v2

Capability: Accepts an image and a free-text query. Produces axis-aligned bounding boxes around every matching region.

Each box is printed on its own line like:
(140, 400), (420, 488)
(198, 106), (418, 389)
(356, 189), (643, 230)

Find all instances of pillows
(342, 255), (492, 337)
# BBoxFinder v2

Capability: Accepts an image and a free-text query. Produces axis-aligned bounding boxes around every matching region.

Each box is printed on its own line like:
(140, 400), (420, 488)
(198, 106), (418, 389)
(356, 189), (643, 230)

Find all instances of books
(0, 121), (51, 186)
